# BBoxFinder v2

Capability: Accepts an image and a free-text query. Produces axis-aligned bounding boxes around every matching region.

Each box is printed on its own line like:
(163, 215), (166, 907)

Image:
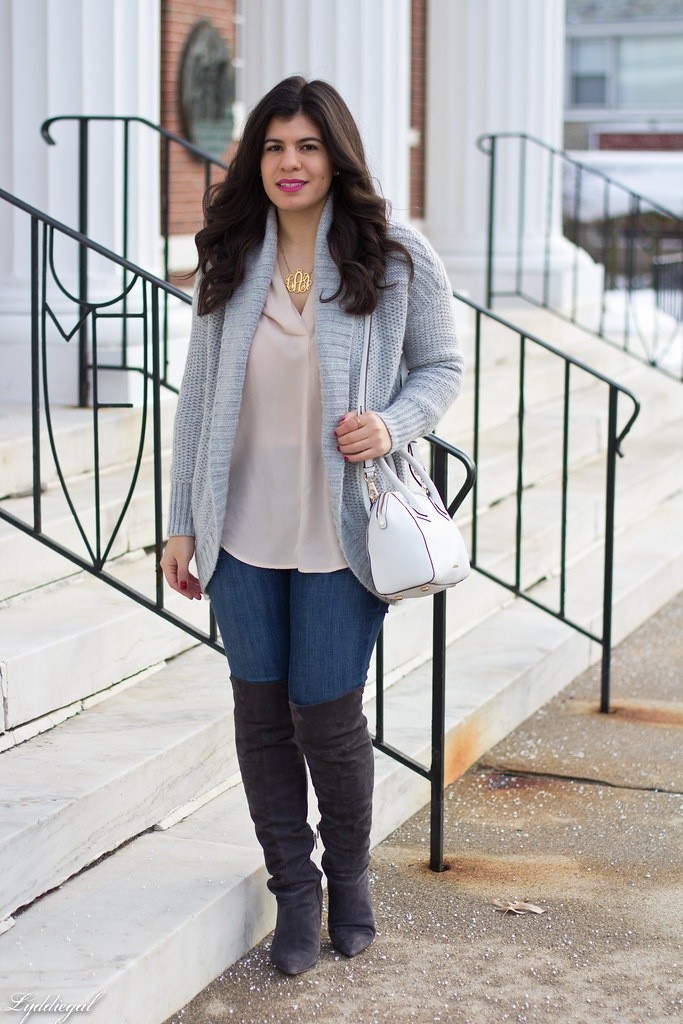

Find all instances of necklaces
(277, 243), (317, 293)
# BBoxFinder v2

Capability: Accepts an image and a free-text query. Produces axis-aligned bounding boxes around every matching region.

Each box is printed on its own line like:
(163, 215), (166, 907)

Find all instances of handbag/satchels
(365, 490), (470, 600)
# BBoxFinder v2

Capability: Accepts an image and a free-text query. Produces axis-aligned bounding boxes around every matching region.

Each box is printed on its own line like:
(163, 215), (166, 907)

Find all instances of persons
(158, 77), (462, 975)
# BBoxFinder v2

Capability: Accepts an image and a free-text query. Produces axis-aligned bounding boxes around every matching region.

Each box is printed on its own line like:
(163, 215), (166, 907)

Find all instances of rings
(354, 417), (362, 430)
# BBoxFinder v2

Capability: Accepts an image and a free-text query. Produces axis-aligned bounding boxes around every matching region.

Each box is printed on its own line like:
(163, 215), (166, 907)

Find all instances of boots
(229, 674), (323, 975)
(289, 688), (376, 956)
